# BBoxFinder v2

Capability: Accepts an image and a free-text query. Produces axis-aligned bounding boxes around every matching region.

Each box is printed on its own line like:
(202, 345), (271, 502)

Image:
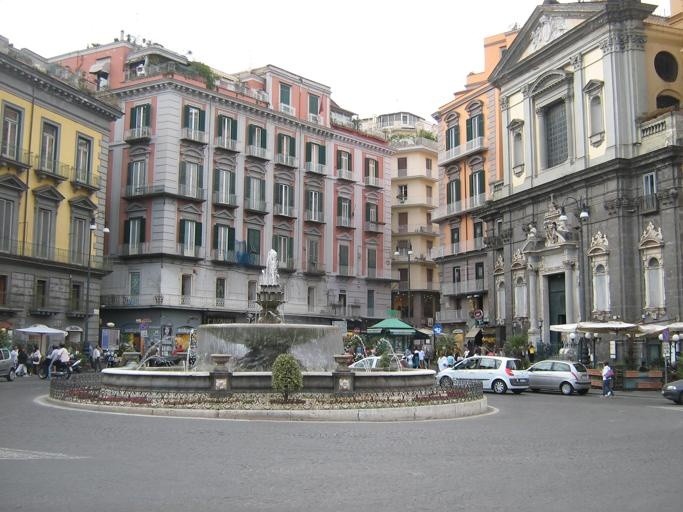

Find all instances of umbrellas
(15, 323), (68, 353)
(549, 318), (683, 342)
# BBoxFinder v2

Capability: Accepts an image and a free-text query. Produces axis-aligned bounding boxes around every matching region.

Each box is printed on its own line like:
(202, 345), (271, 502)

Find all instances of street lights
(584, 331), (601, 368)
(538, 317), (543, 342)
(105, 321), (116, 346)
(82, 215), (112, 359)
(657, 332), (679, 384)
(556, 194), (589, 323)
(392, 239), (415, 323)
(568, 332), (584, 364)
(160, 323), (174, 357)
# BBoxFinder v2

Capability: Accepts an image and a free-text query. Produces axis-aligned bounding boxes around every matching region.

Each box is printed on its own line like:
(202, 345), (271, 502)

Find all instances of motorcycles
(37, 351), (75, 380)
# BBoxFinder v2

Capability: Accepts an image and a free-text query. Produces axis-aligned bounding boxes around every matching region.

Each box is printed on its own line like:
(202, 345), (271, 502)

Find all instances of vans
(0, 347), (16, 381)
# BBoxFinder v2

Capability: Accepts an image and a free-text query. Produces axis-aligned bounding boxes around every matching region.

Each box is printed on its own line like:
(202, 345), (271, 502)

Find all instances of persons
(92, 344), (103, 372)
(162, 326), (170, 340)
(44, 345), (59, 380)
(29, 346), (41, 376)
(57, 343), (70, 363)
(638, 361), (649, 371)
(402, 345), (494, 372)
(11, 345), (19, 369)
(527, 345), (535, 363)
(14, 348), (28, 377)
(602, 360), (611, 397)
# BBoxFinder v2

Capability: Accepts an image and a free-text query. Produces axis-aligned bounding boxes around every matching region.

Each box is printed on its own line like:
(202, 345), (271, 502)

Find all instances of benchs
(584, 369), (664, 392)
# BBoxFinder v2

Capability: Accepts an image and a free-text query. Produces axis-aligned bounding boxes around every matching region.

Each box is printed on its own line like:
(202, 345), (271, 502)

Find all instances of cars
(349, 354), (592, 397)
(658, 378), (683, 405)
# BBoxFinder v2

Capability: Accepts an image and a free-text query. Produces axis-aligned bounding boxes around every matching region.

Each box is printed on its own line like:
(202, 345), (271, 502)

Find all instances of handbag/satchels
(605, 370), (614, 378)
